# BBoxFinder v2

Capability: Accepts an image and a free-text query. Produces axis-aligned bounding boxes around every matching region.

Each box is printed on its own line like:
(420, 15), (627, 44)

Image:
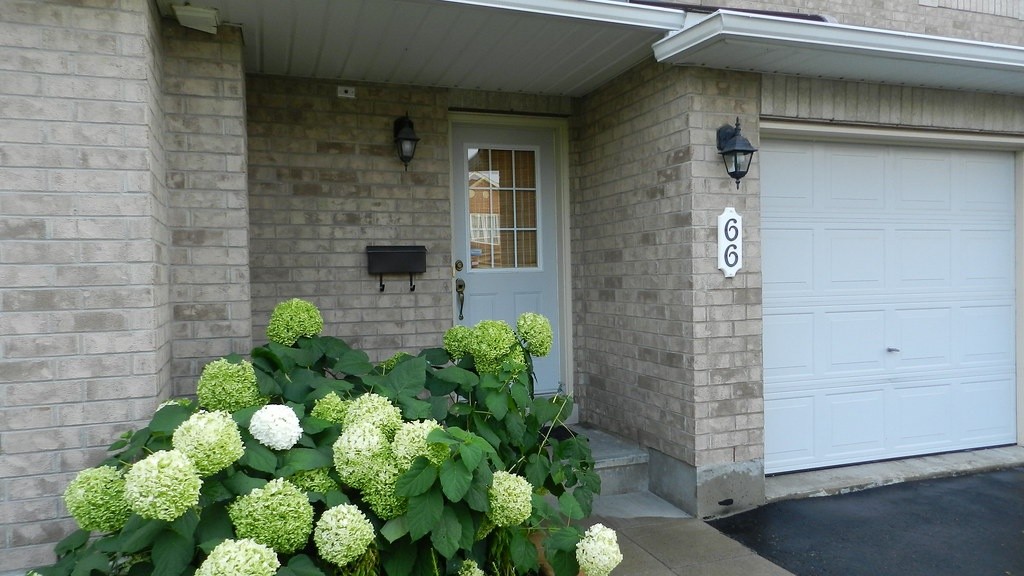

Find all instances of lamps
(715, 117), (758, 190)
(392, 112), (421, 174)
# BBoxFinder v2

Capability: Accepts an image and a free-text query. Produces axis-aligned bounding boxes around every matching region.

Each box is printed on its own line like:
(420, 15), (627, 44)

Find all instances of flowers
(25, 298), (622, 576)
